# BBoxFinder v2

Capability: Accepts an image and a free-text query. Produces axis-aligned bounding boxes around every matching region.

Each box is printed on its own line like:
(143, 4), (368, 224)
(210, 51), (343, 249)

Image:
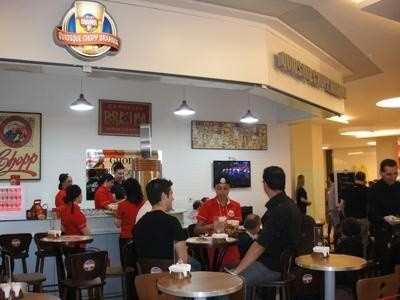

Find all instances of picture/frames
(98, 99), (152, 136)
(0, 111), (42, 180)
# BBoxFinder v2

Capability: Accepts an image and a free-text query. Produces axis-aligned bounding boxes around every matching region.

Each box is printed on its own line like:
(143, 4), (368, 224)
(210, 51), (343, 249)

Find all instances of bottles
(81, 208), (105, 217)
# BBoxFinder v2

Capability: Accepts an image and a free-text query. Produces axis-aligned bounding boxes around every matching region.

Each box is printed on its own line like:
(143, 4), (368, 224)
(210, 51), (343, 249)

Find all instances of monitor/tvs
(213, 160), (251, 188)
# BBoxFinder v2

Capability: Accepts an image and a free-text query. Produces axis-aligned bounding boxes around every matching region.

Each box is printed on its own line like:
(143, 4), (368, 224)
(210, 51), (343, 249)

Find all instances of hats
(213, 173), (232, 189)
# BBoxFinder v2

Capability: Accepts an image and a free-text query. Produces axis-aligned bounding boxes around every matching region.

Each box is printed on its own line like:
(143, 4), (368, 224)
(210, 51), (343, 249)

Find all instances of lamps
(240, 93), (258, 123)
(69, 75), (94, 111)
(174, 85), (196, 116)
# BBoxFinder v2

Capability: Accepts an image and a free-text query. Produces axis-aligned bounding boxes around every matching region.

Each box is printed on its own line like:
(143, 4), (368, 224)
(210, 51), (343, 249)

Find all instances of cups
(49, 230), (61, 239)
(0, 282), (26, 300)
(212, 216), (239, 238)
(74, 0), (105, 50)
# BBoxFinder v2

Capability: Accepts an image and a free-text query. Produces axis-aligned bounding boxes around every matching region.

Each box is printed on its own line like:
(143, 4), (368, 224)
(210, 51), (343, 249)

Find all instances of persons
(216, 165), (303, 300)
(236, 212), (262, 259)
(94, 174), (124, 210)
(61, 184), (93, 300)
(325, 158), (400, 276)
(194, 175), (243, 271)
(132, 178), (202, 273)
(135, 177), (153, 225)
(114, 178), (145, 300)
(111, 164), (126, 201)
(296, 174), (312, 214)
(54, 173), (73, 208)
